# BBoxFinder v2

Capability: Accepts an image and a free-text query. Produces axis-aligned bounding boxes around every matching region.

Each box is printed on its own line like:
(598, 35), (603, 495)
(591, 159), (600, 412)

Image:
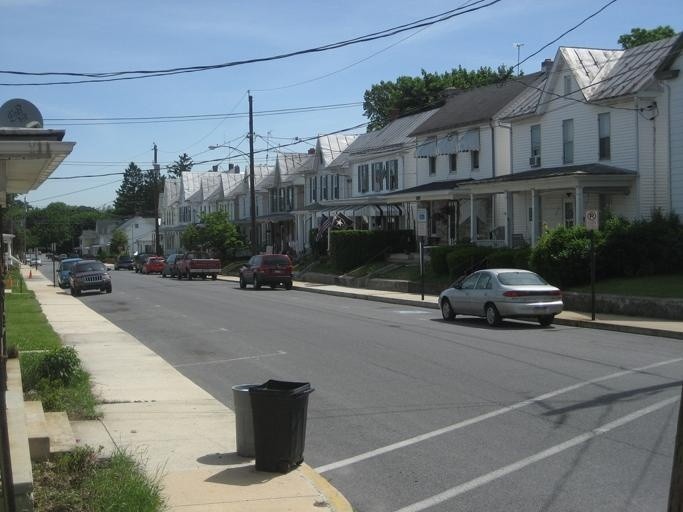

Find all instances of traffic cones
(27, 270), (32, 279)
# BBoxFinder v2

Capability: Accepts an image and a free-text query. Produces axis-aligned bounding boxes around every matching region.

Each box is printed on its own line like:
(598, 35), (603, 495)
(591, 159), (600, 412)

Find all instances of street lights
(207, 144), (258, 253)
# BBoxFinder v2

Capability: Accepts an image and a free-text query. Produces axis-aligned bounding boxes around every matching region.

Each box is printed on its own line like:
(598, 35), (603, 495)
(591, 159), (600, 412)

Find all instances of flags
(332, 212), (354, 230)
(315, 214), (331, 241)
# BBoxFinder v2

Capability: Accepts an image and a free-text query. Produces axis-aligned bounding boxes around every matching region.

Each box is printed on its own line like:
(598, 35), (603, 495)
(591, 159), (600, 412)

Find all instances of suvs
(67, 259), (113, 297)
(238, 253), (294, 291)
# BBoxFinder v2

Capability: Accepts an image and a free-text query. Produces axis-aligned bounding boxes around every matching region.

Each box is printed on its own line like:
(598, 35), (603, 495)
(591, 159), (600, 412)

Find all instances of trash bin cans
(231, 378), (315, 474)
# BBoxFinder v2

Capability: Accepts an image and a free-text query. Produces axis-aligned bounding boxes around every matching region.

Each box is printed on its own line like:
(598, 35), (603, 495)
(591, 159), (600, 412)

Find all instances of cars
(436, 268), (565, 327)
(113, 252), (221, 281)
(56, 258), (86, 289)
(16, 250), (67, 266)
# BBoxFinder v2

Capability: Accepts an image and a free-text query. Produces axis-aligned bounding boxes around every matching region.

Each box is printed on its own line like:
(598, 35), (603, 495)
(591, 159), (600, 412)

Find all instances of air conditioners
(529, 156), (540, 167)
